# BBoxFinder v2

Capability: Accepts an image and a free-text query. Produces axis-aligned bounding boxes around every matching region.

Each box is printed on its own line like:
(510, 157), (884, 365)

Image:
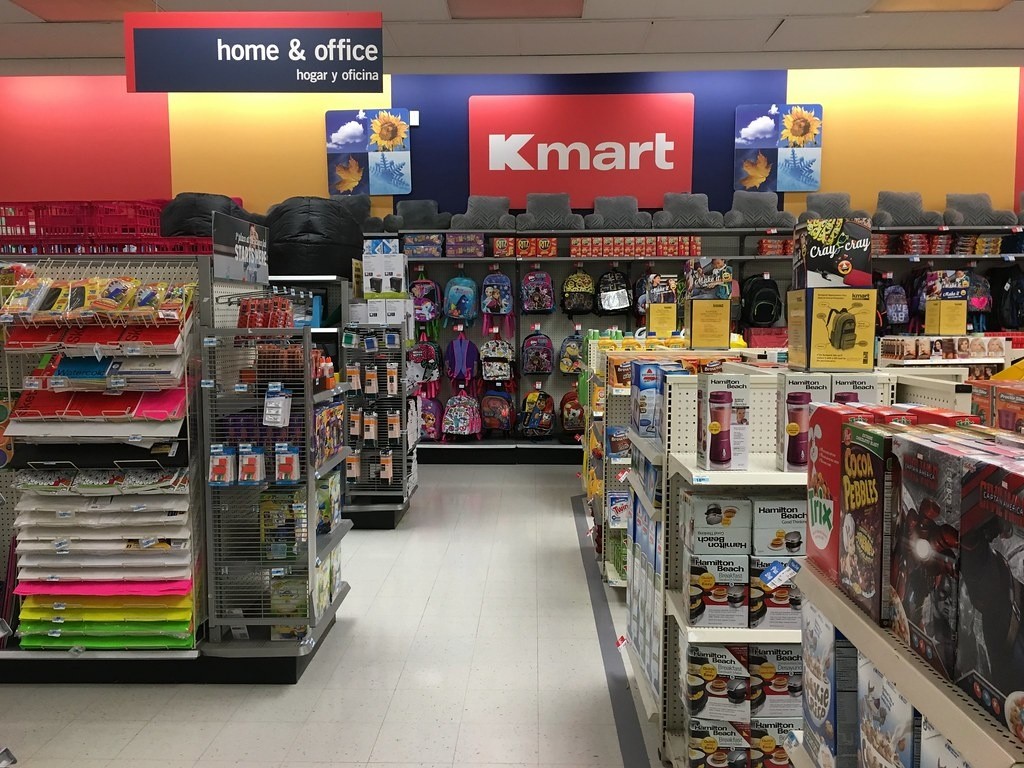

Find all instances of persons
(906, 338), (1004, 356)
(968, 365), (996, 380)
(649, 259), (732, 304)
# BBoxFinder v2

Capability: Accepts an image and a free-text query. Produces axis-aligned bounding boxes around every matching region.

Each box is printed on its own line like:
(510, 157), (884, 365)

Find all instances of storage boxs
(2, 199), (213, 255)
(233, 215), (1024, 768)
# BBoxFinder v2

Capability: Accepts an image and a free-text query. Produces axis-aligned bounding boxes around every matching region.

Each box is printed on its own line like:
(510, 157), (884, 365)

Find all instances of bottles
(708, 391), (732, 464)
(583, 329), (624, 365)
(834, 392), (859, 404)
(785, 392), (813, 467)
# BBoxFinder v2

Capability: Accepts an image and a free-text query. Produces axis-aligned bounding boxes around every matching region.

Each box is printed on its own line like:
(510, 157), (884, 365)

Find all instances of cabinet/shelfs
(0, 255), (353, 684)
(624, 361), (972, 768)
(267, 275), (419, 529)
(397, 228), (1024, 468)
(581, 339), (666, 516)
(784, 556), (1024, 768)
(596, 350), (767, 587)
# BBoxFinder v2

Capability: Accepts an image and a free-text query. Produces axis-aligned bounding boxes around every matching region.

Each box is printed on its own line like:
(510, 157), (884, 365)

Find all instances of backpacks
(875, 265), (1024, 333)
(824, 308), (856, 350)
(406, 265), (793, 443)
